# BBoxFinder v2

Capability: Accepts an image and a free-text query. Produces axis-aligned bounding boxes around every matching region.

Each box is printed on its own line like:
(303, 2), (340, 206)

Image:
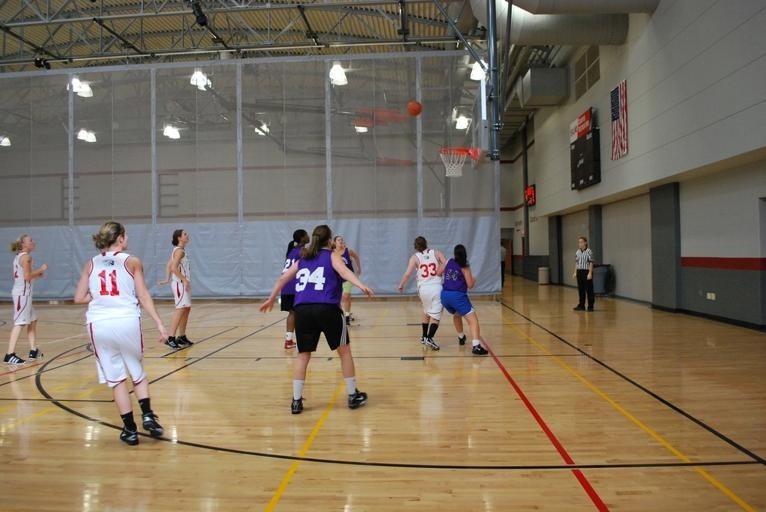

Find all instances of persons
(573, 237), (595, 311)
(332, 235), (361, 326)
(279, 229), (310, 349)
(158, 228), (195, 349)
(3, 234), (49, 365)
(258, 224), (376, 414)
(501, 243), (507, 287)
(72, 221), (174, 445)
(435, 244), (488, 355)
(398, 236), (446, 350)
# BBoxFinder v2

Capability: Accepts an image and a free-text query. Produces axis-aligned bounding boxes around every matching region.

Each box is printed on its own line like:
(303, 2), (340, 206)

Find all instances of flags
(610, 79), (628, 162)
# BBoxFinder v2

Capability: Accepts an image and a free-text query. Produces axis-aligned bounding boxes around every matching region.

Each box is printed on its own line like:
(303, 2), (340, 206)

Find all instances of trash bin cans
(591, 263), (611, 296)
(538, 267), (549, 285)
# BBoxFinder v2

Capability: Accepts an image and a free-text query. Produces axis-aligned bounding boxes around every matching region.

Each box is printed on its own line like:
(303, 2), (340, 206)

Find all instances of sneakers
(177, 335), (193, 346)
(285, 339), (296, 349)
(142, 412), (164, 434)
(29, 349), (43, 362)
(3, 352), (25, 364)
(348, 388), (367, 408)
(121, 423), (139, 445)
(419, 336), (440, 350)
(472, 344), (488, 354)
(291, 396), (304, 414)
(457, 334), (466, 345)
(166, 336), (178, 348)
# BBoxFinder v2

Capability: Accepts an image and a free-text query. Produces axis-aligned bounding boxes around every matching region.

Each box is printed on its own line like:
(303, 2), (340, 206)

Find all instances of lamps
(469, 59), (489, 82)
(190, 67), (212, 91)
(76, 127), (97, 143)
(329, 61), (348, 86)
(66, 74), (95, 98)
(163, 122), (181, 139)
(32, 56), (53, 73)
(1, 132), (12, 147)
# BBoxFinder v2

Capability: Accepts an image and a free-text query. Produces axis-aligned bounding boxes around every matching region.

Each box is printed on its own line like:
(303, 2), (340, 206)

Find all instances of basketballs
(406, 99), (421, 116)
